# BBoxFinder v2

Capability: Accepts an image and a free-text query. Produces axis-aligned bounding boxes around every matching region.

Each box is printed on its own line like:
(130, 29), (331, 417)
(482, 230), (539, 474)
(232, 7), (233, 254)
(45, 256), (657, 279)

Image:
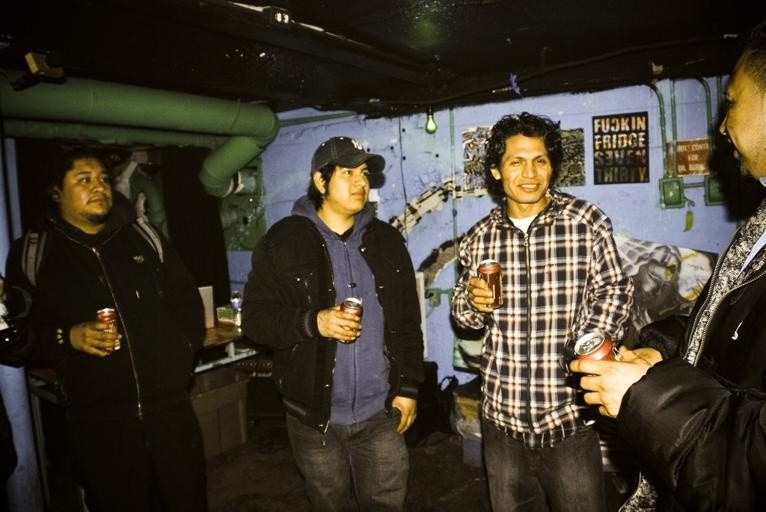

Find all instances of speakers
(251, 7), (293, 42)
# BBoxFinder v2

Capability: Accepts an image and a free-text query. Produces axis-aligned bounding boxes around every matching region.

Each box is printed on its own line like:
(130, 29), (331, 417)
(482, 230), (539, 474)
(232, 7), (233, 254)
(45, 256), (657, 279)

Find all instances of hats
(311, 136), (385, 175)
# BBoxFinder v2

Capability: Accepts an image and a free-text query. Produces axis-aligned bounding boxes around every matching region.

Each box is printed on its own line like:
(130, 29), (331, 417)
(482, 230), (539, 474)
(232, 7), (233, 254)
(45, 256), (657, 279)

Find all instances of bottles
(229, 290), (245, 327)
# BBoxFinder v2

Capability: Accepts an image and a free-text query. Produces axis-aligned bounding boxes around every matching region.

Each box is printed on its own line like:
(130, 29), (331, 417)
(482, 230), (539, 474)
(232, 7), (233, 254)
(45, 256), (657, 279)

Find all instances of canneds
(573, 331), (618, 363)
(476, 261), (504, 309)
(337, 299), (363, 344)
(95, 308), (121, 352)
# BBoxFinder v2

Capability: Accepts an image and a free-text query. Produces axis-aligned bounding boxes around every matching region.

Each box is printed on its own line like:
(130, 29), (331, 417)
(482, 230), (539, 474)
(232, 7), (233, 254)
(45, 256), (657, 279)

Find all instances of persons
(0, 147), (205, 512)
(449, 112), (634, 512)
(570, 40), (766, 512)
(241, 136), (425, 512)
